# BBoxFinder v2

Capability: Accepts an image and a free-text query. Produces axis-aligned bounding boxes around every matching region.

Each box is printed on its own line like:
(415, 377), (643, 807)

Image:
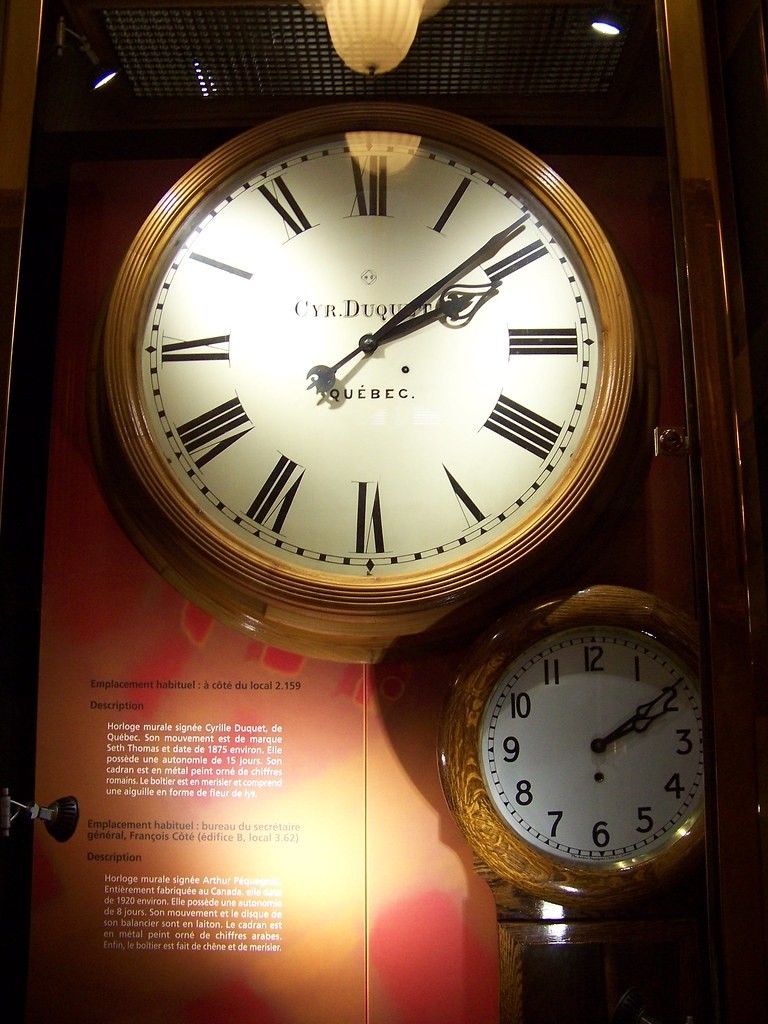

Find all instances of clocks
(437, 585), (723, 1024)
(84, 100), (660, 664)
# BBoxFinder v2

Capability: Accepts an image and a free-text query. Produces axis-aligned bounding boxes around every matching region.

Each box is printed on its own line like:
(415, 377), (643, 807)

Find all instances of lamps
(56, 17), (119, 91)
(317, 0), (427, 78)
(590, 0), (622, 37)
